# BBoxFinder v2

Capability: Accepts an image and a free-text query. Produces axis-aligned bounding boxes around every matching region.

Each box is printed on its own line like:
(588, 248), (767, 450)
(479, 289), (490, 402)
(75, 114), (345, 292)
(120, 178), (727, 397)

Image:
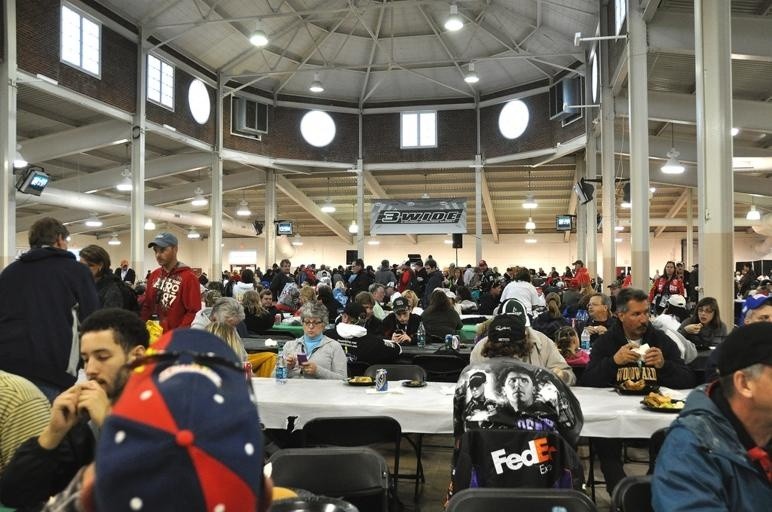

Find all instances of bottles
(453, 335), (461, 351)
(581, 327), (590, 355)
(375, 369), (388, 391)
(275, 347), (288, 385)
(576, 309), (582, 321)
(445, 334), (453, 350)
(417, 321), (426, 348)
(582, 310), (589, 321)
(275, 314), (281, 326)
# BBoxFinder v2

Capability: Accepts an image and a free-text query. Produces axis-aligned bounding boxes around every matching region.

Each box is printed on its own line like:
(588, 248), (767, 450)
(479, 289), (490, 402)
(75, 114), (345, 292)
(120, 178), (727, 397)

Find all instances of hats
(741, 293), (771, 324)
(667, 295), (689, 310)
(692, 264), (698, 268)
(676, 261), (685, 267)
(414, 260), (423, 267)
(367, 266), (375, 273)
(147, 233), (179, 248)
(96, 329), (265, 511)
(338, 303), (367, 321)
(392, 297), (409, 312)
(490, 280), (501, 288)
(468, 372), (487, 388)
(445, 292), (456, 300)
(316, 283), (326, 291)
(317, 287), (334, 300)
(489, 314), (526, 342)
(498, 297), (531, 330)
(432, 287), (444, 292)
(750, 279), (761, 287)
(479, 261), (487, 267)
(393, 264), (399, 267)
(556, 281), (564, 289)
(539, 281), (546, 288)
(760, 278), (772, 286)
(335, 282), (344, 289)
(712, 322), (772, 378)
(321, 277), (331, 283)
(387, 281), (398, 290)
(572, 260), (584, 267)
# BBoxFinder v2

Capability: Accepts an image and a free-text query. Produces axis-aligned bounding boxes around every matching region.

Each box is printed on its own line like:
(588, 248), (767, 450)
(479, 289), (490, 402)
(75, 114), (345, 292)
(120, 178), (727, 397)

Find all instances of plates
(641, 399), (687, 413)
(613, 383), (660, 394)
(348, 378), (377, 386)
(401, 380), (427, 387)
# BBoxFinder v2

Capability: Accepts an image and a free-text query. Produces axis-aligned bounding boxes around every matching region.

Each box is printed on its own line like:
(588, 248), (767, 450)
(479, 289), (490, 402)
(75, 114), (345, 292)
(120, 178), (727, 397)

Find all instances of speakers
(453, 234), (462, 248)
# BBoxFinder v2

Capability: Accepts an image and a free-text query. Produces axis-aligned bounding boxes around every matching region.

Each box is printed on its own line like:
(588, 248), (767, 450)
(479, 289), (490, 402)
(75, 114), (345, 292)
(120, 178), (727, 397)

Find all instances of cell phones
(395, 330), (403, 334)
(297, 354), (307, 366)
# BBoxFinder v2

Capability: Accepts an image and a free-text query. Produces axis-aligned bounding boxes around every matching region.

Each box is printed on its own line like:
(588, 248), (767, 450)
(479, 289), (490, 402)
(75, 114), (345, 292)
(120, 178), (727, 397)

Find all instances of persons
(333, 281), (346, 302)
(140, 233), (201, 334)
(532, 281), (545, 307)
(495, 366), (558, 431)
(1, 308), (150, 511)
(261, 263), (278, 280)
(317, 286), (344, 323)
(239, 290), (274, 334)
(0, 217), (98, 406)
(294, 267), (299, 275)
(690, 263), (698, 299)
(533, 293), (570, 342)
(233, 269), (257, 299)
(493, 267), (501, 279)
(296, 265), (309, 288)
(652, 321), (772, 512)
(383, 298), (425, 344)
(564, 266), (573, 289)
(469, 299), (576, 390)
(206, 290), (222, 307)
(571, 261), (591, 289)
(481, 283), (501, 315)
(653, 294), (686, 331)
(366, 266), (374, 275)
(331, 266), (344, 288)
(464, 372), (503, 430)
(385, 281), (402, 306)
(497, 268), (537, 327)
(744, 294), (771, 322)
(402, 290), (417, 313)
(449, 268), (465, 299)
(223, 270), (241, 297)
(424, 260), (443, 308)
(551, 271), (560, 281)
(580, 288), (696, 497)
(316, 264), (331, 280)
(733, 264), (772, 299)
(336, 292), (383, 329)
(295, 286), (318, 317)
(574, 293), (617, 348)
(80, 245), (133, 312)
(448, 316), (583, 488)
(575, 296), (589, 320)
(204, 297), (248, 363)
(270, 259), (293, 301)
(260, 289), (285, 321)
(198, 274), (208, 294)
(421, 290), (463, 343)
(391, 263), (399, 279)
(207, 282), (224, 297)
(534, 369), (577, 431)
(76, 331), (272, 512)
(369, 283), (387, 322)
(454, 286), (477, 314)
(135, 286), (147, 303)
(549, 267), (555, 278)
(651, 261), (685, 302)
(115, 260), (136, 287)
(677, 261), (689, 299)
(190, 290), (222, 330)
(323, 303), (396, 365)
(414, 261), (427, 283)
(146, 270), (152, 280)
(555, 326), (590, 365)
(475, 260), (495, 291)
(428, 255), (432, 261)
(503, 268), (513, 282)
(320, 272), (332, 287)
(1, 369), (52, 511)
(543, 278), (561, 298)
(270, 300), (348, 380)
(401, 264), (410, 292)
(677, 298), (726, 350)
(375, 260), (397, 286)
(347, 259), (370, 296)
(464, 264), (475, 287)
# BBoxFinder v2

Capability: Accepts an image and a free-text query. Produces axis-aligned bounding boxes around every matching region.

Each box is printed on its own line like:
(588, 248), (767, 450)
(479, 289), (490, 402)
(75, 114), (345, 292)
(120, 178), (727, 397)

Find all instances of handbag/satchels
(225, 280), (233, 298)
(433, 345), (461, 356)
(332, 287), (347, 305)
(276, 283), (301, 313)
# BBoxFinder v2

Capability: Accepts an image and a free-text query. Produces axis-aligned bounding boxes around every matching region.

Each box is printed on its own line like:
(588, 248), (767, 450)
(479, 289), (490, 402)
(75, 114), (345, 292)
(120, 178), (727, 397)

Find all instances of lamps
(249, 18), (268, 47)
(523, 167), (539, 209)
(237, 200), (251, 216)
(253, 222), (264, 236)
(292, 233), (303, 245)
(307, 70), (325, 92)
(349, 219), (358, 234)
(464, 60), (479, 83)
(443, 0), (464, 32)
(187, 230), (201, 238)
(525, 209), (536, 230)
(659, 121), (686, 175)
(192, 195), (209, 205)
(746, 196), (761, 219)
(525, 230), (537, 244)
(108, 237), (122, 245)
(117, 177), (133, 190)
(321, 198), (337, 213)
(145, 220), (156, 230)
(86, 216), (103, 227)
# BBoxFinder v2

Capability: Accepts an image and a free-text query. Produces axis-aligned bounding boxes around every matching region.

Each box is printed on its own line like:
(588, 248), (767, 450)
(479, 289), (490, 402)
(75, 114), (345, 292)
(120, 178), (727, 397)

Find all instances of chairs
(610, 475), (655, 512)
(446, 488), (598, 512)
(356, 446), (358, 446)
(414, 354), (461, 382)
(265, 452), (390, 512)
(572, 365), (634, 501)
(364, 365), (426, 498)
(303, 416), (402, 502)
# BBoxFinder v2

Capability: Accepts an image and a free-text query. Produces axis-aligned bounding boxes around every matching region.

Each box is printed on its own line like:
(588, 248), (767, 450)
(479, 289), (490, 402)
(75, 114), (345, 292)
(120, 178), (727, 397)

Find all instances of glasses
(698, 306), (715, 316)
(350, 264), (359, 268)
(585, 302), (603, 309)
(425, 267), (432, 270)
(455, 271), (460, 273)
(302, 319), (322, 328)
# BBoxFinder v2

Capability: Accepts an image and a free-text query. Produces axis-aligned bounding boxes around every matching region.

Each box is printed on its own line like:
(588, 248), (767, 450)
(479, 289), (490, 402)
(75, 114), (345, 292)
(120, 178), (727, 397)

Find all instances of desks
(239, 336), (560, 375)
(77, 368), (702, 437)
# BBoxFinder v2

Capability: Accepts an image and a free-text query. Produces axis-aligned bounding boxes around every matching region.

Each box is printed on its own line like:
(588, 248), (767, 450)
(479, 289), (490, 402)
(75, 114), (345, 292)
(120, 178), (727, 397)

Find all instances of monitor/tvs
(574, 177), (602, 205)
(278, 223), (292, 235)
(18, 172), (48, 195)
(556, 214), (577, 231)
